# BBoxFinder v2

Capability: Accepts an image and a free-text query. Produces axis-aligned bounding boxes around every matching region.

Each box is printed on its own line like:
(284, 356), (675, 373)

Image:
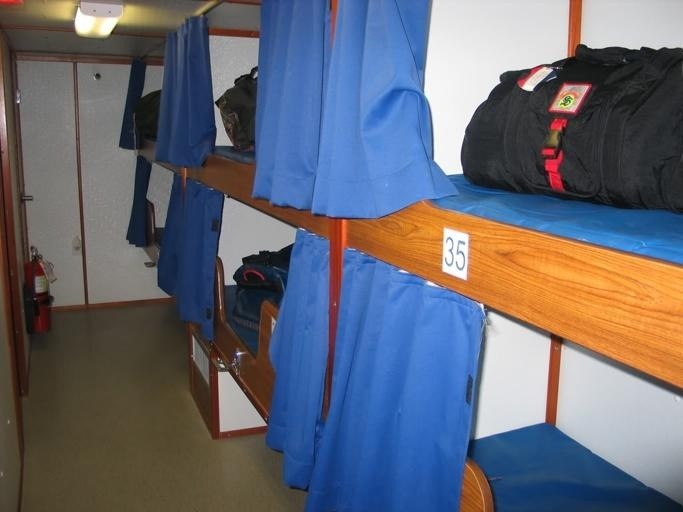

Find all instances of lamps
(74, 0), (122, 40)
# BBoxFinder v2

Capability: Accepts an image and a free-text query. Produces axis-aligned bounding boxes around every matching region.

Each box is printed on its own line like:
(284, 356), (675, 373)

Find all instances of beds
(118, 1), (683, 508)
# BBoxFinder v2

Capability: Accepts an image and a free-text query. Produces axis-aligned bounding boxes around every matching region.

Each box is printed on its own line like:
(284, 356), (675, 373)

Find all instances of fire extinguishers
(26, 246), (53, 334)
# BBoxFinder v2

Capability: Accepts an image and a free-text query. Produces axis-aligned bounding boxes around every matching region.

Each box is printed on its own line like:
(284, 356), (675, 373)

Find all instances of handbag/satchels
(231, 242), (294, 295)
(460, 44), (682, 214)
(214, 67), (257, 153)
